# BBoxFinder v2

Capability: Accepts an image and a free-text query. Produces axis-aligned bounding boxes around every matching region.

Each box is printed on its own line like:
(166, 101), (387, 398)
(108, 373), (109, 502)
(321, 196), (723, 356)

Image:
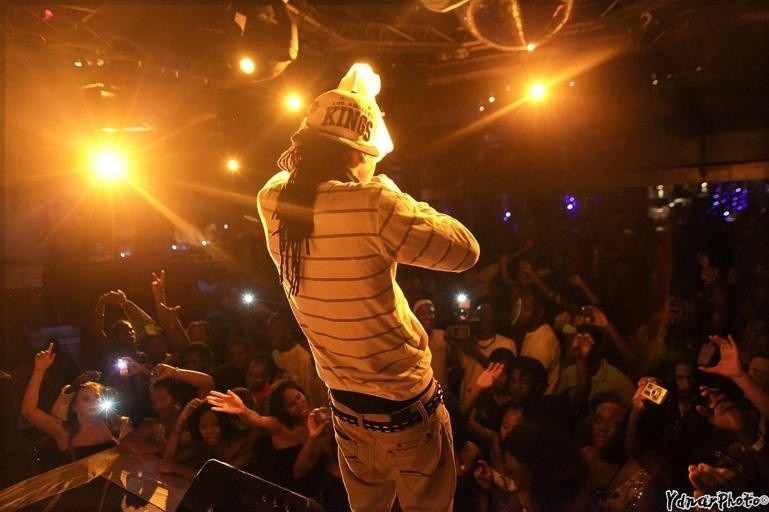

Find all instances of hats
(305, 88), (382, 158)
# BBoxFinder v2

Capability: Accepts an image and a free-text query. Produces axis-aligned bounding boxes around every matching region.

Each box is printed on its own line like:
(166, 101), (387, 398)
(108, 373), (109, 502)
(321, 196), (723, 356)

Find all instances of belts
(329, 383), (445, 433)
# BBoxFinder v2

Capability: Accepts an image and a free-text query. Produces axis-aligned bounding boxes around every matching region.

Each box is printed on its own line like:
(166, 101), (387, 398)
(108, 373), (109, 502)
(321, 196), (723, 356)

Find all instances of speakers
(174, 459), (327, 512)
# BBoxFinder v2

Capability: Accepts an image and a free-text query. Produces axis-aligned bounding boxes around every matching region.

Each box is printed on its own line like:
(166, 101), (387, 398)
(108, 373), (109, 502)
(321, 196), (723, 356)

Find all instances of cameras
(108, 291), (122, 303)
(315, 408), (331, 423)
(641, 381), (669, 406)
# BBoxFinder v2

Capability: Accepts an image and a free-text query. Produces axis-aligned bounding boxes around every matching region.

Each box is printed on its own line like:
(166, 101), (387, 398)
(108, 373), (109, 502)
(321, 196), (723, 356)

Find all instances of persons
(253, 62), (483, 511)
